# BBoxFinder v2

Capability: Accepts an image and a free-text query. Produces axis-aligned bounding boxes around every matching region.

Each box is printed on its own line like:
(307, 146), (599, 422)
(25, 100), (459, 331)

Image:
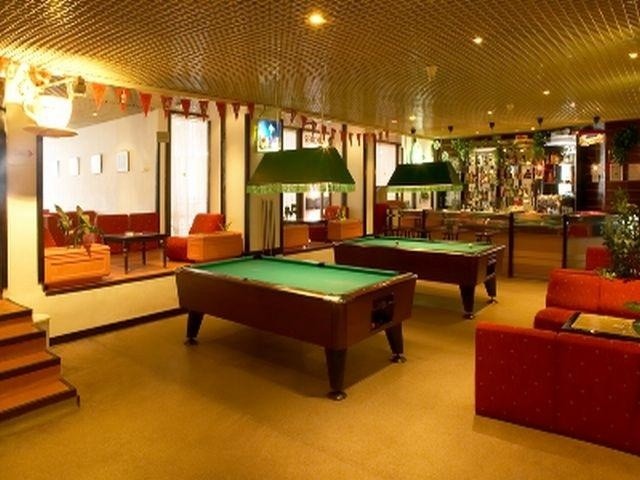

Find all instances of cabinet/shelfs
(433, 124), (610, 211)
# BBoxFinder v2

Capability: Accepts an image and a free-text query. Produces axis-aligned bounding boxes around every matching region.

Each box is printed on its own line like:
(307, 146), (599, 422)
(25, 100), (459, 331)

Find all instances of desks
(327, 218), (362, 242)
(283, 224), (309, 253)
(186, 231), (244, 262)
(102, 231), (170, 274)
(44, 243), (112, 288)
(333, 234), (506, 320)
(176, 251), (418, 401)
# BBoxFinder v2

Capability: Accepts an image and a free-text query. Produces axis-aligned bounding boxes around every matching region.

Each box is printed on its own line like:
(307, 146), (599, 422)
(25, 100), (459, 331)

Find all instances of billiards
(467, 243), (473, 249)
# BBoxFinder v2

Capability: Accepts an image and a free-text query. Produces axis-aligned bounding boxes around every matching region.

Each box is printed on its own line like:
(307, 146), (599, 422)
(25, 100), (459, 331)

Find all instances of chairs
(309, 219), (328, 242)
(166, 214), (225, 263)
(474, 245), (640, 459)
(385, 210), (501, 244)
(42, 210), (159, 252)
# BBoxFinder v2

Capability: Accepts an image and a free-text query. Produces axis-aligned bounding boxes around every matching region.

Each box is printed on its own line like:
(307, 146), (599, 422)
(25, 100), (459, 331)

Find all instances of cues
(261, 199), (276, 257)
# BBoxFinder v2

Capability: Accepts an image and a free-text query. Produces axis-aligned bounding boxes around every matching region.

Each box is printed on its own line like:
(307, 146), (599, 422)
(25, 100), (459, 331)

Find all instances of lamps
(384, 66), (465, 193)
(247, 0), (355, 193)
